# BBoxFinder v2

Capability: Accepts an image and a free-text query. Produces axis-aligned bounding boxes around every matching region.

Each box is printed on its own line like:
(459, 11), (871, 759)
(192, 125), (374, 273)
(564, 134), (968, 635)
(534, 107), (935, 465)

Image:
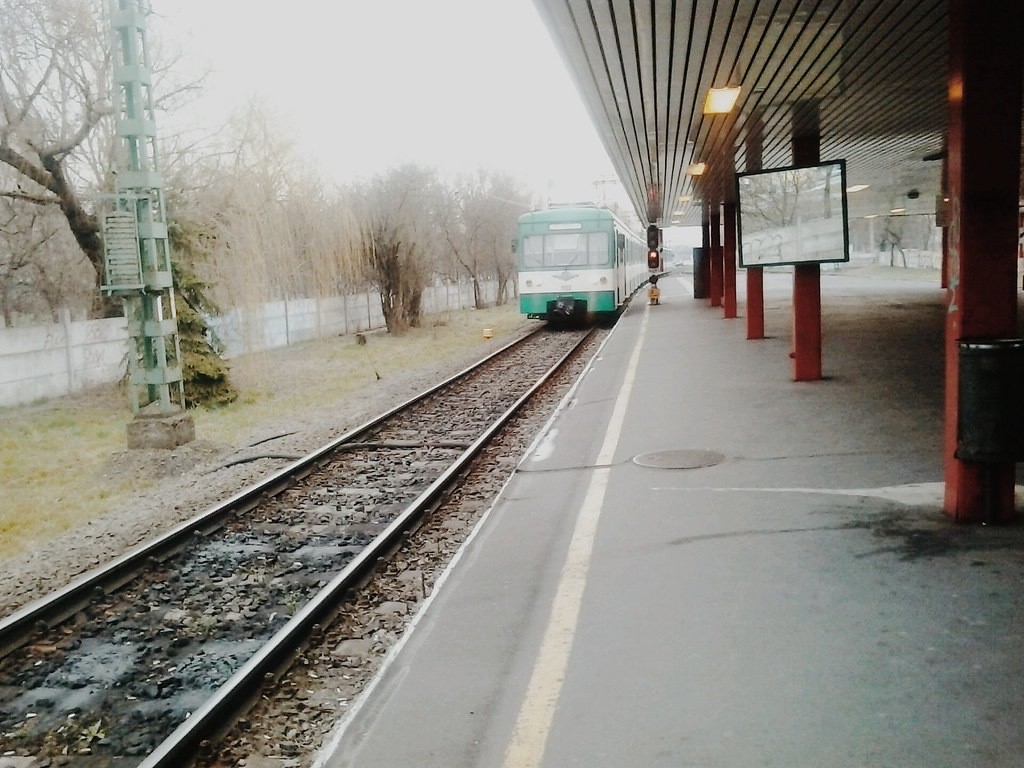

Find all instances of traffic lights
(648, 250), (660, 270)
(647, 224), (658, 248)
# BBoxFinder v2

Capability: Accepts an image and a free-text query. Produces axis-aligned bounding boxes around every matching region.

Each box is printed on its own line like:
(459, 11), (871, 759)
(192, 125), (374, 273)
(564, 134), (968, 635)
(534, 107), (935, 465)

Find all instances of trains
(511, 201), (675, 322)
